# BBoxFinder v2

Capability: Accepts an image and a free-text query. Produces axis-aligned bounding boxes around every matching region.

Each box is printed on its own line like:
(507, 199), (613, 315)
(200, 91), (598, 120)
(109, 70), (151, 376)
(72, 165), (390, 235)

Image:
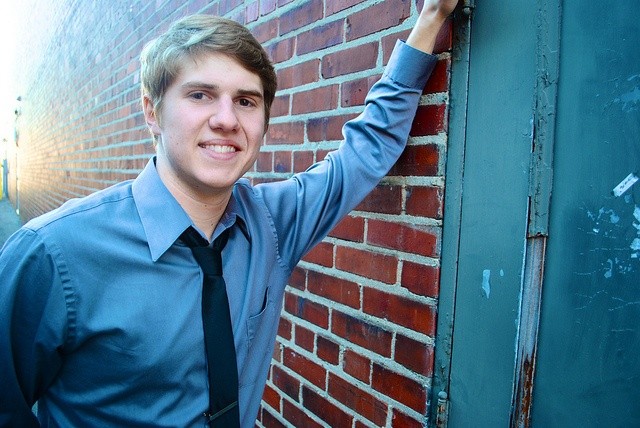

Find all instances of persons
(0, 0), (463, 428)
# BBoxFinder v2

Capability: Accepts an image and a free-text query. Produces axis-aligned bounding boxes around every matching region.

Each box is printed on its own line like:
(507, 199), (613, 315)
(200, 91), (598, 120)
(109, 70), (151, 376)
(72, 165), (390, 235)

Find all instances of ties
(178, 226), (240, 427)
